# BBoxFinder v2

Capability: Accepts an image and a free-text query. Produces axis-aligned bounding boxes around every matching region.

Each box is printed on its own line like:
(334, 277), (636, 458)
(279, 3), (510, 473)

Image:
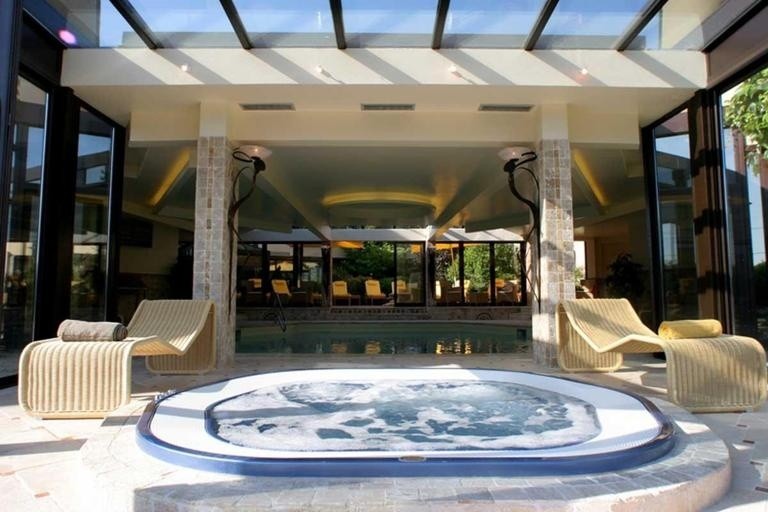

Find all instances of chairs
(496, 285), (519, 303)
(249, 278), (412, 306)
(17, 297), (217, 419)
(556, 297), (768, 414)
(439, 276), (462, 303)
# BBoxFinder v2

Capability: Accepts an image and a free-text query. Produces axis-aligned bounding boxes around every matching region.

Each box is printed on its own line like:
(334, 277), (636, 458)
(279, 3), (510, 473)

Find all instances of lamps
(497, 145), (540, 234)
(221, 144), (274, 247)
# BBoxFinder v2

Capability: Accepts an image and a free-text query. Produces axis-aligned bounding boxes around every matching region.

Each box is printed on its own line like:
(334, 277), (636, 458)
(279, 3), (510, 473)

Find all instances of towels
(655, 317), (724, 340)
(56, 316), (128, 342)
